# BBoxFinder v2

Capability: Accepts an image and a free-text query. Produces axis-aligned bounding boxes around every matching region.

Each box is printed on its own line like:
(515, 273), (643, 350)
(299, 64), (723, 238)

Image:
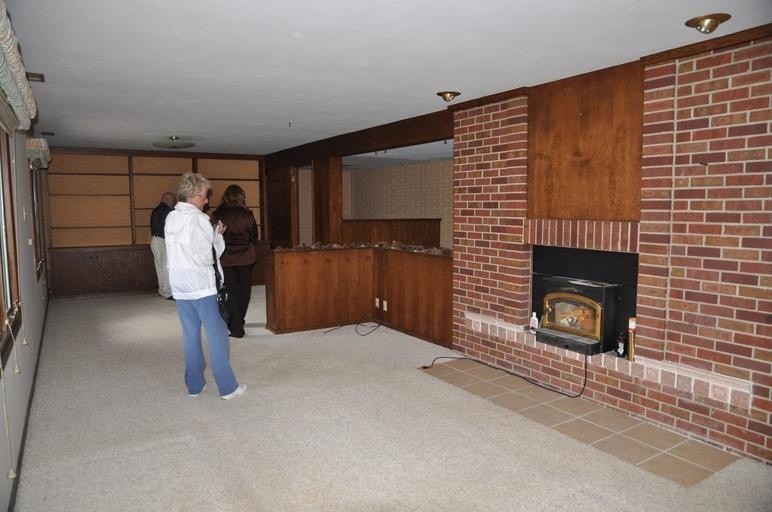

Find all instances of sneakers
(188, 381), (209, 398)
(221, 382), (247, 400)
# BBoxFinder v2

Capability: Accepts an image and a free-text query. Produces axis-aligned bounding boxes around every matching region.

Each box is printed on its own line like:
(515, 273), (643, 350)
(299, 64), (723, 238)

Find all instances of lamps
(685, 14), (732, 35)
(436, 91), (460, 102)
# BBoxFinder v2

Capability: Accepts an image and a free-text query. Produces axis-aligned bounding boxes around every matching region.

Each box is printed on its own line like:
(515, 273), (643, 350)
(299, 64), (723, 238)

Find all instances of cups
(628, 327), (635, 361)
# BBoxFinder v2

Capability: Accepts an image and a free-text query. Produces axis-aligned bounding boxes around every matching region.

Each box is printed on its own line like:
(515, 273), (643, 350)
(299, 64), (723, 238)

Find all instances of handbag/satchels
(218, 285), (233, 323)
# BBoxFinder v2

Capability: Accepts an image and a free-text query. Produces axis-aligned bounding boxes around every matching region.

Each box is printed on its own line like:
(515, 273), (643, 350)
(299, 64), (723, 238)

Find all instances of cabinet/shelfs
(266, 244), (374, 333)
(373, 248), (452, 350)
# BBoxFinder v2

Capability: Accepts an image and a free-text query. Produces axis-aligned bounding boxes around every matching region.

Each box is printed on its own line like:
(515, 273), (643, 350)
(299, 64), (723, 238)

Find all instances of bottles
(529, 312), (538, 335)
(616, 332), (628, 357)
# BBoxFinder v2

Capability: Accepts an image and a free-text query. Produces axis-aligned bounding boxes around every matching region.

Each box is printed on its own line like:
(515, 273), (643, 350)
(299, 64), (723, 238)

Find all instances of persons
(212, 184), (270, 338)
(164, 173), (249, 400)
(148, 192), (176, 302)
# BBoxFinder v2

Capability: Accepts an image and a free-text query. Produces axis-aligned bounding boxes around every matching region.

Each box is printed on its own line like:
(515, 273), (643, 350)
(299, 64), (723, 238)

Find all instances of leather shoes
(227, 318), (246, 338)
(166, 295), (176, 300)
